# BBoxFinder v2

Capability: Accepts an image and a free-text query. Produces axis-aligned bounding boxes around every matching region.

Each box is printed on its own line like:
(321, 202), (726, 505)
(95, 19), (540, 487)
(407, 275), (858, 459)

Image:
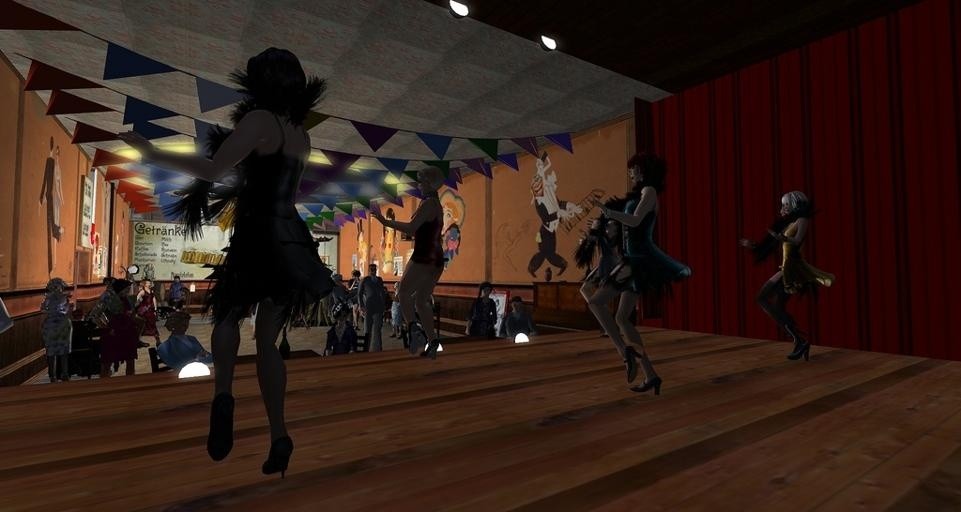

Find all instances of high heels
(406, 322), (422, 353)
(786, 339), (810, 362)
(207, 392), (235, 462)
(622, 346), (643, 384)
(630, 377), (662, 395)
(419, 339), (439, 360)
(261, 436), (293, 478)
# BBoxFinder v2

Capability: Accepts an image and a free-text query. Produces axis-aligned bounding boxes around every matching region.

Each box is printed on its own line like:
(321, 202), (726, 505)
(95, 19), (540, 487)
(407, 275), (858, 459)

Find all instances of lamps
(445, 2), (480, 21)
(540, 17), (567, 54)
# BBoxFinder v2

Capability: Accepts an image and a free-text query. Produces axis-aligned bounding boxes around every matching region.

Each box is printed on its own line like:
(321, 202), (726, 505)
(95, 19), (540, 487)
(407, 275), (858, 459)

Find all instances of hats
(111, 278), (133, 295)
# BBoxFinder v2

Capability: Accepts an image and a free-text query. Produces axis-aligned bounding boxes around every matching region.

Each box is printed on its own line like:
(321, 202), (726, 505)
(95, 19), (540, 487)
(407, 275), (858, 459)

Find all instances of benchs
(519, 276), (648, 336)
(375, 291), (484, 340)
(165, 289), (216, 315)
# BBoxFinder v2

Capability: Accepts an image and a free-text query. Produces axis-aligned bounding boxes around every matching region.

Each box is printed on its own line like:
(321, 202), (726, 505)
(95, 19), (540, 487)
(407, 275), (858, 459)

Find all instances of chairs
(149, 344), (189, 373)
